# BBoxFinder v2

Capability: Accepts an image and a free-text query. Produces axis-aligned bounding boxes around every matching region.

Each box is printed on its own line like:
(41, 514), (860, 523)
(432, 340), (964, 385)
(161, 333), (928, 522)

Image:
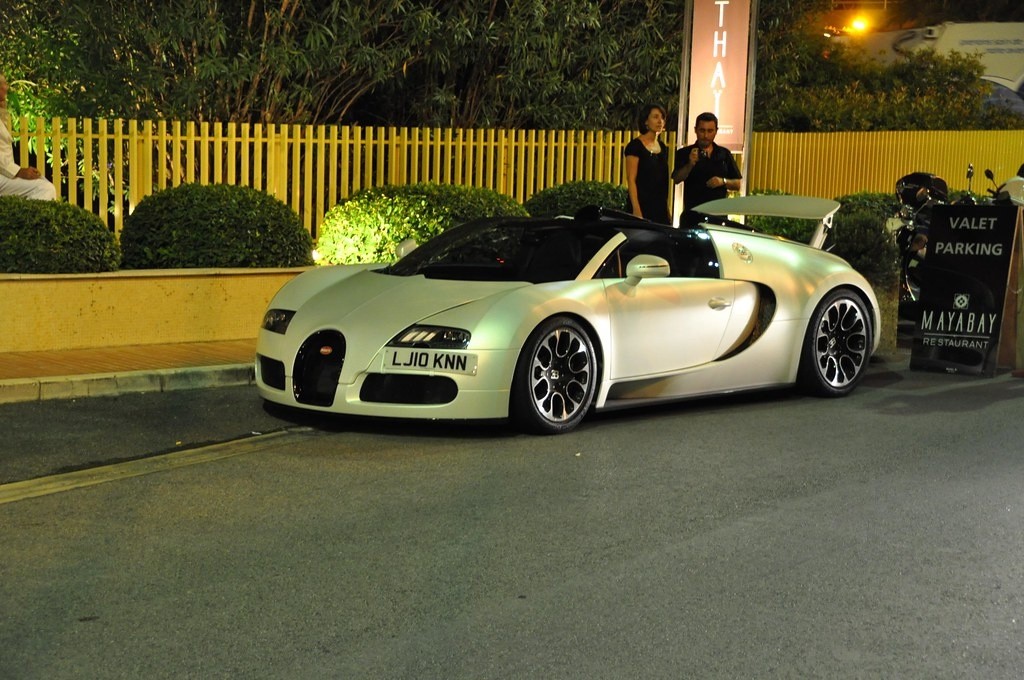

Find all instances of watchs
(723, 177), (728, 185)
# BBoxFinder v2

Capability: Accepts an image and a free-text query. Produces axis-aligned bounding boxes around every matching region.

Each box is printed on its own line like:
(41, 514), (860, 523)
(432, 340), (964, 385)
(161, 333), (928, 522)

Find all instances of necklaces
(642, 135), (657, 155)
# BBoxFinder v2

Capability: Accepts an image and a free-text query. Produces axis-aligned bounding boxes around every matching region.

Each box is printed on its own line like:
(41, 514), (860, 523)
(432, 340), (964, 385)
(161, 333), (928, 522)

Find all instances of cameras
(698, 150), (708, 160)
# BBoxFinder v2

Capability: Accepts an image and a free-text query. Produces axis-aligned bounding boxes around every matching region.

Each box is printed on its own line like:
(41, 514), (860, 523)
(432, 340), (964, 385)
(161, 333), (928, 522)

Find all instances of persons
(623, 102), (672, 227)
(0, 71), (59, 200)
(670, 112), (743, 227)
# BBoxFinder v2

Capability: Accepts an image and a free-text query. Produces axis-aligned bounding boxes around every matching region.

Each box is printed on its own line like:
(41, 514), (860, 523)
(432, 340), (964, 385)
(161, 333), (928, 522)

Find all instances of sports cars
(253, 194), (884, 437)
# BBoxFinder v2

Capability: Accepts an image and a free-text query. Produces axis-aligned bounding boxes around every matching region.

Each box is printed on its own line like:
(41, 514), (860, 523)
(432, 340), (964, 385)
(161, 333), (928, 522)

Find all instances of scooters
(886, 164), (978, 322)
